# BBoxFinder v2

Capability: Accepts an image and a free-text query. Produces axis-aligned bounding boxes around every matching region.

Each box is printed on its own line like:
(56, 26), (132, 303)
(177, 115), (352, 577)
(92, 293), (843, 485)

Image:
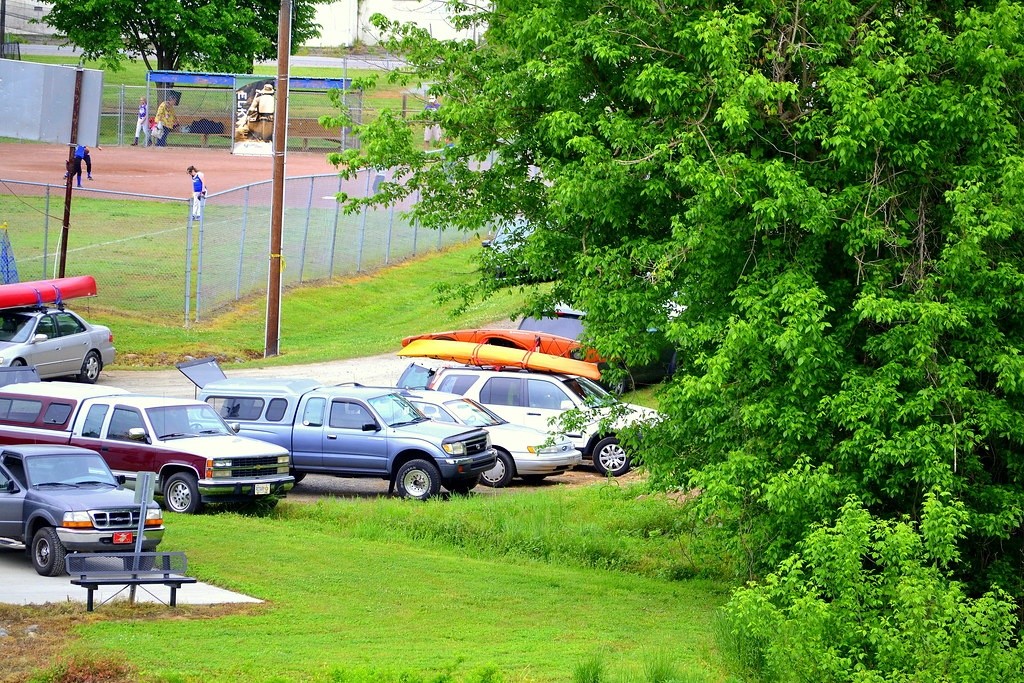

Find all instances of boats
(397, 328), (607, 381)
(0, 276), (98, 307)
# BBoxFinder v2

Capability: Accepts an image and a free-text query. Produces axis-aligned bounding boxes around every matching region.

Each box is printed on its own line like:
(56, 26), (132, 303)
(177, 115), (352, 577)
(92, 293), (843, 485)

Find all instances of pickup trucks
(0, 366), (296, 515)
(397, 363), (675, 478)
(177, 358), (499, 501)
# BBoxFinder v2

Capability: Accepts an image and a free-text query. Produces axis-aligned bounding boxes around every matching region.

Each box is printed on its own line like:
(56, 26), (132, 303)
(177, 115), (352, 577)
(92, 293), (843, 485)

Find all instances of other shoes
(132, 142), (137, 146)
(87, 176), (92, 179)
(148, 141), (152, 146)
(192, 216), (195, 220)
(196, 216), (200, 220)
(64, 174), (68, 179)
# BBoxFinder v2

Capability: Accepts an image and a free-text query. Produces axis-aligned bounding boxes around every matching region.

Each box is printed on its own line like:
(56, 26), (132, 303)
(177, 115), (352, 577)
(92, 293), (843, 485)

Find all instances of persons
(131, 97), (151, 146)
(444, 137), (455, 148)
(424, 97), (441, 151)
(63, 145), (102, 188)
(247, 84), (274, 141)
(187, 166), (207, 220)
(155, 96), (179, 147)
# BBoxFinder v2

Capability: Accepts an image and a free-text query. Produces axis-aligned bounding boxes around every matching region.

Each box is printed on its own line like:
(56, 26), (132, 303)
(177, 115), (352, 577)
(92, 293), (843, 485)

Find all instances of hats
(260, 84), (275, 93)
(186, 166), (194, 173)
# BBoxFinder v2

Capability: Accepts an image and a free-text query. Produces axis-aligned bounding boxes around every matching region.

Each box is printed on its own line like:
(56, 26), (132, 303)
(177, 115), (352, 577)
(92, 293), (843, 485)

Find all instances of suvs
(0, 444), (164, 577)
(520, 303), (680, 397)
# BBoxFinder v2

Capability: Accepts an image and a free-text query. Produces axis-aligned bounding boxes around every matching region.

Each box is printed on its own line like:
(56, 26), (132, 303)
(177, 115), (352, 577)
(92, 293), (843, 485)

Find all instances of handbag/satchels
(151, 125), (165, 139)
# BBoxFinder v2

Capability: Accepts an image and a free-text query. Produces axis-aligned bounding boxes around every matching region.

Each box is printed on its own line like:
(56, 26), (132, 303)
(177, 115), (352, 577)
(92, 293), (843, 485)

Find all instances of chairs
(349, 403), (361, 416)
(424, 406), (436, 417)
(36, 324), (54, 339)
(2, 321), (17, 334)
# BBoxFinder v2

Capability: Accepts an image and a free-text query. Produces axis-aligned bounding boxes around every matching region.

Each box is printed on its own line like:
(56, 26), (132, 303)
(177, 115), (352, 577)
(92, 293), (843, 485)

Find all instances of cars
(0, 307), (115, 382)
(490, 215), (556, 284)
(396, 387), (582, 488)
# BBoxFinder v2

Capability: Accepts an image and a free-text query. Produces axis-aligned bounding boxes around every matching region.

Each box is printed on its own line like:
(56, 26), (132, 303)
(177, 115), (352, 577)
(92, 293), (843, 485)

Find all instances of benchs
(289, 136), (342, 151)
(153, 131), (231, 147)
(64, 553), (198, 613)
